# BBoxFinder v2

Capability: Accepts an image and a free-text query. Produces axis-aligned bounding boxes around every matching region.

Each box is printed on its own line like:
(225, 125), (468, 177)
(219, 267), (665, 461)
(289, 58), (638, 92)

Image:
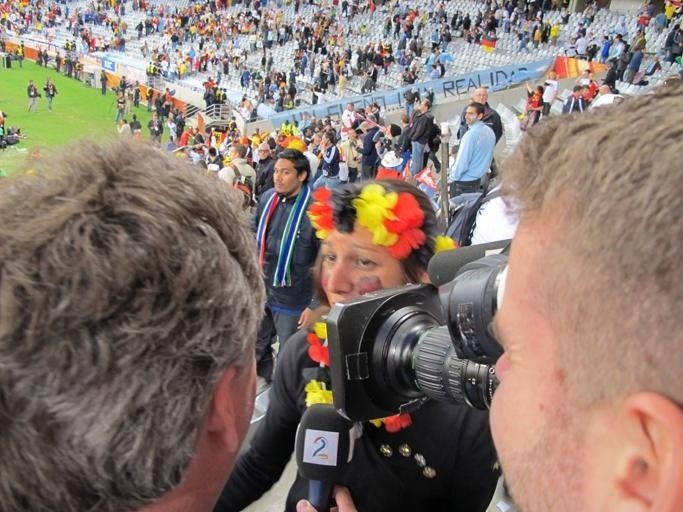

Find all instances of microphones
(296, 403), (355, 512)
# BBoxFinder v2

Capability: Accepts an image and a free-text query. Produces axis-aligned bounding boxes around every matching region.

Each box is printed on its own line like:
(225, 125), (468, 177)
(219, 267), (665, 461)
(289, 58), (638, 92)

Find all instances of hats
(380, 150), (403, 168)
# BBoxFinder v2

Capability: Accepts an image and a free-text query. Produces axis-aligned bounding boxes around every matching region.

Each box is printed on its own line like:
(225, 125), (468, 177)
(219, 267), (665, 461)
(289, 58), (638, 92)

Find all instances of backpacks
(229, 163), (255, 211)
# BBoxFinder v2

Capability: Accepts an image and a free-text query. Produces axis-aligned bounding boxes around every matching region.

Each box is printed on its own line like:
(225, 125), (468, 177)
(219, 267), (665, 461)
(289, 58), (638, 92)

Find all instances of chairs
(1, 1), (682, 124)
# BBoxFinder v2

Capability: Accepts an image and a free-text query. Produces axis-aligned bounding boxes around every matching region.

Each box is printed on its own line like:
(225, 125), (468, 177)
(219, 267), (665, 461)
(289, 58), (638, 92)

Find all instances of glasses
(257, 149), (268, 153)
